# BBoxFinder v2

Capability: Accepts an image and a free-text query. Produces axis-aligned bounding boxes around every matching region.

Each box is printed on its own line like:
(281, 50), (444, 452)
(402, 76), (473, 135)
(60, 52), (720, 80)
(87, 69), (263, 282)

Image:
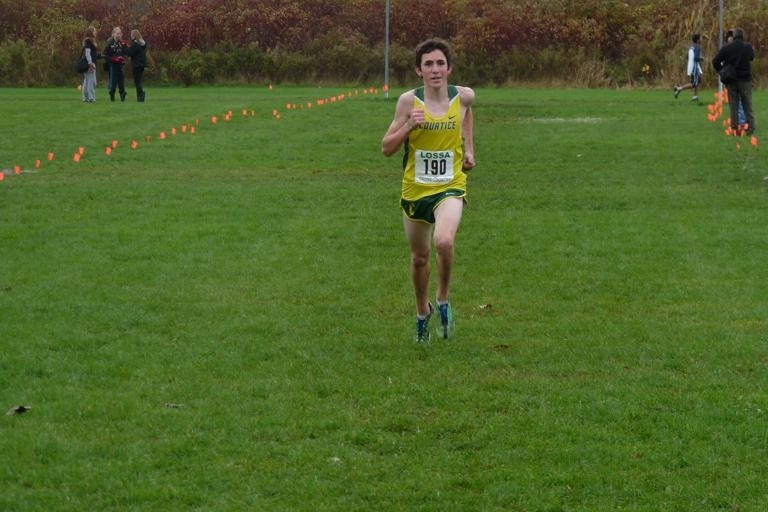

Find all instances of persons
(712, 27), (755, 135)
(123, 29), (148, 102)
(380, 39), (476, 343)
(724, 28), (747, 127)
(674, 34), (704, 103)
(104, 27), (127, 101)
(81, 26), (106, 103)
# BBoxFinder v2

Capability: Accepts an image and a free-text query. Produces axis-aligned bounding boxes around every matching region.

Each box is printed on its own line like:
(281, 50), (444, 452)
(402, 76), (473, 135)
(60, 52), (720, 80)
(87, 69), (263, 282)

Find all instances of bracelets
(407, 119), (414, 132)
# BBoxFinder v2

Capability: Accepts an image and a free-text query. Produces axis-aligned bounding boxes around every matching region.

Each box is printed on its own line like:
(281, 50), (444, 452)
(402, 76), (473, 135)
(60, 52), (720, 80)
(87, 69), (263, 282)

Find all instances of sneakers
(415, 301), (435, 346)
(434, 297), (453, 341)
(672, 82), (682, 101)
